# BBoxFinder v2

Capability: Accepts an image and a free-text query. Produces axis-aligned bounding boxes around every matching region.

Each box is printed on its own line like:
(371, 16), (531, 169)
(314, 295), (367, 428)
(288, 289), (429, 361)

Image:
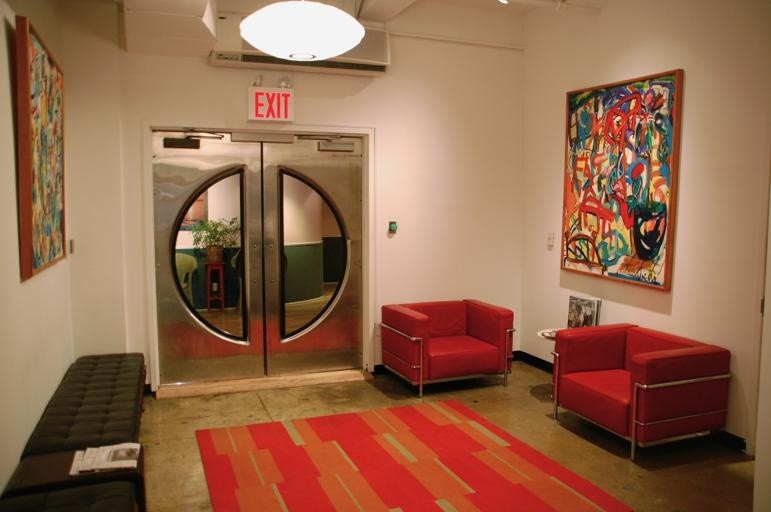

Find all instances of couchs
(549, 323), (732, 462)
(0, 481), (136, 512)
(381, 299), (516, 399)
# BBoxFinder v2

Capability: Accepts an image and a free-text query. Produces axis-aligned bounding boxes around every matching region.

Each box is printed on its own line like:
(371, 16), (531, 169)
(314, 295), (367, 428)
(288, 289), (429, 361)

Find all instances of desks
(2, 444), (147, 512)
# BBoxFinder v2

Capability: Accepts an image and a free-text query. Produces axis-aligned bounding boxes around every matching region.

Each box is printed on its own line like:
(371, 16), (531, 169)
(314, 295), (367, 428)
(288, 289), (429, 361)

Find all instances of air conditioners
(208, 12), (391, 77)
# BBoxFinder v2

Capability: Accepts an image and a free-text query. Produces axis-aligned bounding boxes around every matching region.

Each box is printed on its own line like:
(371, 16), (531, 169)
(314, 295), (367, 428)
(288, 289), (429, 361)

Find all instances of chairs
(176, 253), (197, 308)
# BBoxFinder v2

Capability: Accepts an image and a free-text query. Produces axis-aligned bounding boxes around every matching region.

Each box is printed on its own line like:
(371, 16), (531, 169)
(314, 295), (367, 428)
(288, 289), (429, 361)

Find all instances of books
(567, 295), (602, 329)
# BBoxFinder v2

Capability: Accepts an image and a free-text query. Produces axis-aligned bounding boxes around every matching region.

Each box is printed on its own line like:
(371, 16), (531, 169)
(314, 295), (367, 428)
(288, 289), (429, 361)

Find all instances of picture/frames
(14, 14), (68, 283)
(559, 68), (684, 294)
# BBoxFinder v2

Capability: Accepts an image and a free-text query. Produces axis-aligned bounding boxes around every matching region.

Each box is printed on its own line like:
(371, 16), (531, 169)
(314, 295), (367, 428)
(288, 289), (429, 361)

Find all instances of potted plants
(190, 218), (242, 261)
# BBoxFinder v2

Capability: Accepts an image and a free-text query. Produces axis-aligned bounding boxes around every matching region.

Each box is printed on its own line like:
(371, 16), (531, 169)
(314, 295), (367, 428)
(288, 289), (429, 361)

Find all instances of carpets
(196, 399), (636, 512)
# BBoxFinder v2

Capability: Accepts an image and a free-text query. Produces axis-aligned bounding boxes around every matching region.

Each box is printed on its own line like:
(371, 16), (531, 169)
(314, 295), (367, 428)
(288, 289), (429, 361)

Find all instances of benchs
(20, 353), (147, 460)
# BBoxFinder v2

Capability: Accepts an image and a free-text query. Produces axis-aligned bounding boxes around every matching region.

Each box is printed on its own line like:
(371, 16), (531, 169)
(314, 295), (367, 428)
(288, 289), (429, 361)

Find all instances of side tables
(537, 329), (565, 401)
